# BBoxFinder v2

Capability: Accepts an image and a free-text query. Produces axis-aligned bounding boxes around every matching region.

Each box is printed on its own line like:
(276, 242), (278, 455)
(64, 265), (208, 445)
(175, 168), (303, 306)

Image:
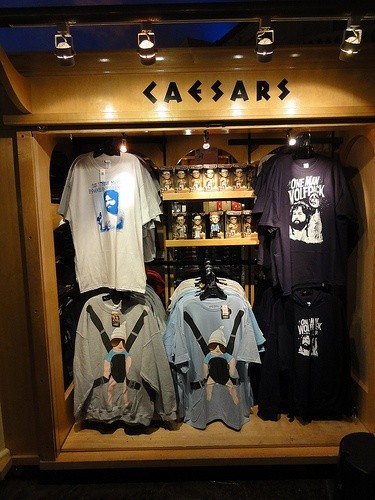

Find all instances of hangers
(293, 134), (319, 162)
(197, 258), (227, 302)
(103, 285), (132, 301)
(91, 136), (121, 159)
(298, 285), (314, 297)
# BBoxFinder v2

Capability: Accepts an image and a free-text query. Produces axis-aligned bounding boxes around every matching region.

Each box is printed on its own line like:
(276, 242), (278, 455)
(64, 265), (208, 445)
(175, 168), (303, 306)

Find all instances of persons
(161, 169), (254, 240)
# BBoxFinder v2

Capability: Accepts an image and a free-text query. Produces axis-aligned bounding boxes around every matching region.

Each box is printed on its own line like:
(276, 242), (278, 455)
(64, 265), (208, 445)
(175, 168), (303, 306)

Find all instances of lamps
(253, 18), (277, 64)
(286, 129), (312, 151)
(52, 21), (77, 65)
(199, 131), (211, 150)
(119, 133), (128, 154)
(338, 15), (364, 63)
(133, 21), (158, 66)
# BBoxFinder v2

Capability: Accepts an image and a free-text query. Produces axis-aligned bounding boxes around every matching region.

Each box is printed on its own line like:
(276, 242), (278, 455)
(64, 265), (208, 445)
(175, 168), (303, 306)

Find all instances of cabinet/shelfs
(15, 122), (375, 472)
